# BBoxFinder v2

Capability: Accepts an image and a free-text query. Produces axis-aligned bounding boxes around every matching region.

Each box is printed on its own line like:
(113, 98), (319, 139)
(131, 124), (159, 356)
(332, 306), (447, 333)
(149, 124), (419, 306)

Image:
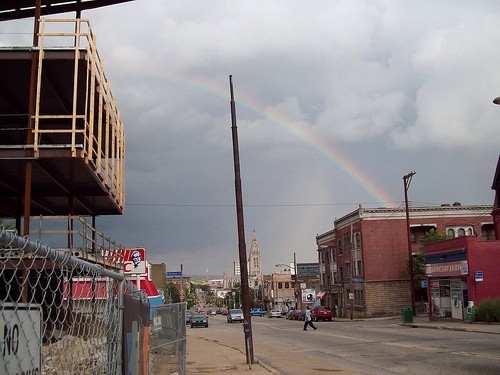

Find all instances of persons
(303, 306), (318, 331)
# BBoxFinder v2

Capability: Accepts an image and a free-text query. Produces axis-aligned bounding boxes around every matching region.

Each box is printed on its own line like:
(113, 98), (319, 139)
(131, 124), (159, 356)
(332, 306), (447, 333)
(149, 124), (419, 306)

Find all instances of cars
(226, 309), (244, 323)
(286, 310), (303, 320)
(268, 310), (282, 318)
(250, 309), (268, 317)
(298, 309), (315, 321)
(186, 303), (228, 317)
(309, 305), (332, 322)
(185, 314), (192, 325)
(188, 313), (209, 328)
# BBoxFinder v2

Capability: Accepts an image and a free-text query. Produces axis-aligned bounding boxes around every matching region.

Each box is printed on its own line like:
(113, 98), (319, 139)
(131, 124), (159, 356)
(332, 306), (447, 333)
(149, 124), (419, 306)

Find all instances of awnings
(317, 292), (325, 299)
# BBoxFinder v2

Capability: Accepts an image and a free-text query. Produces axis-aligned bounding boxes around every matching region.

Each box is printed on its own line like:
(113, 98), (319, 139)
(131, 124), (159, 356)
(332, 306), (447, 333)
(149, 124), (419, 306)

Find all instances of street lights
(274, 251), (298, 310)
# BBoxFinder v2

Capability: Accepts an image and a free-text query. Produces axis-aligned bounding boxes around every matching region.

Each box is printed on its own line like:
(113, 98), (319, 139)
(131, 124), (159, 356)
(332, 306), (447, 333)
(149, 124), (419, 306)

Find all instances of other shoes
(304, 328), (307, 331)
(314, 327), (318, 330)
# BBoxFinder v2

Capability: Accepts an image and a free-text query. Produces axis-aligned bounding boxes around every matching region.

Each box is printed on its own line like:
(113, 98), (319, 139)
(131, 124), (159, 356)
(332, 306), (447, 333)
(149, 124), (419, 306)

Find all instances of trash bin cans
(148, 295), (164, 320)
(401, 307), (413, 323)
